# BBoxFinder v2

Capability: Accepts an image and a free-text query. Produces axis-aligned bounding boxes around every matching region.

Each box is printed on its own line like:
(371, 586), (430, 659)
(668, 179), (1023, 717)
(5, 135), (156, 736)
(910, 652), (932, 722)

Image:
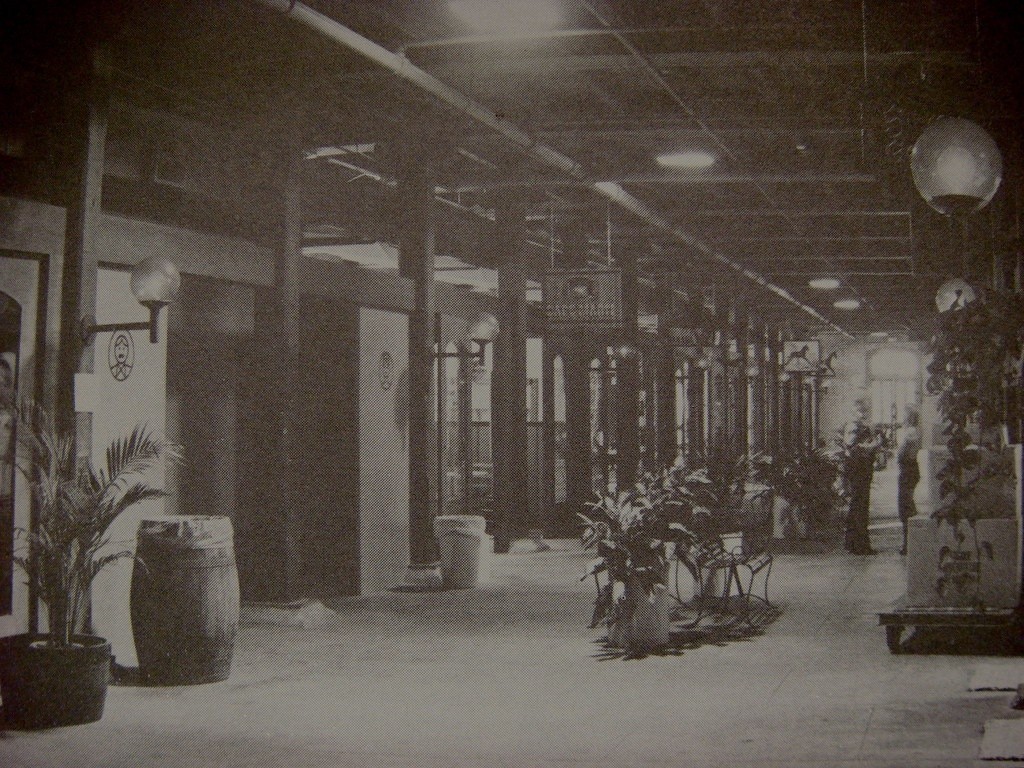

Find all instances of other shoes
(847, 543), (879, 555)
(900, 547), (907, 555)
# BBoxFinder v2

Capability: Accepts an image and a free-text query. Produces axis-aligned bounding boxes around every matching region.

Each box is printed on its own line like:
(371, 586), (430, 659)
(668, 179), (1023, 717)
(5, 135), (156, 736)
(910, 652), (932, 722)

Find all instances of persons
(842, 398), (883, 556)
(896, 406), (921, 556)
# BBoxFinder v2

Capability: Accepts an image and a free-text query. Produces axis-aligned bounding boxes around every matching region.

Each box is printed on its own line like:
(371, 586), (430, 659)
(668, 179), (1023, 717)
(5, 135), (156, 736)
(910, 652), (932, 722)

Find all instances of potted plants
(577, 469), (711, 646)
(1, 385), (188, 728)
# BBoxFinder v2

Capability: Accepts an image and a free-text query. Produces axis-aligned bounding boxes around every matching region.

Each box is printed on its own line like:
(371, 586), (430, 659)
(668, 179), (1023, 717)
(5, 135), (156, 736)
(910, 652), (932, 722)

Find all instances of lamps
(910, 115), (1024, 285)
(434, 311), (498, 366)
(80, 256), (180, 344)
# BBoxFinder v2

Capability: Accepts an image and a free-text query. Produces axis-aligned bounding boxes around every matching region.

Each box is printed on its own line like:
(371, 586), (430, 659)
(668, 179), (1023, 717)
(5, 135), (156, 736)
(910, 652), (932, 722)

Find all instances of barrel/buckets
(130, 516), (240, 685)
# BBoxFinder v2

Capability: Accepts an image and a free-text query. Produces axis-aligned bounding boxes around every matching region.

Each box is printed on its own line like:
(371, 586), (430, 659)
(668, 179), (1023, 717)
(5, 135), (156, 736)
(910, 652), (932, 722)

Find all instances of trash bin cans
(431, 515), (488, 590)
(128, 513), (241, 688)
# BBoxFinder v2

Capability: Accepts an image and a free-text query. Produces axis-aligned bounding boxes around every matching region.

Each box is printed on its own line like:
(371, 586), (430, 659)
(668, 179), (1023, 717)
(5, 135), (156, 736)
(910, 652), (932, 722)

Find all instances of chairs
(685, 528), (773, 630)
